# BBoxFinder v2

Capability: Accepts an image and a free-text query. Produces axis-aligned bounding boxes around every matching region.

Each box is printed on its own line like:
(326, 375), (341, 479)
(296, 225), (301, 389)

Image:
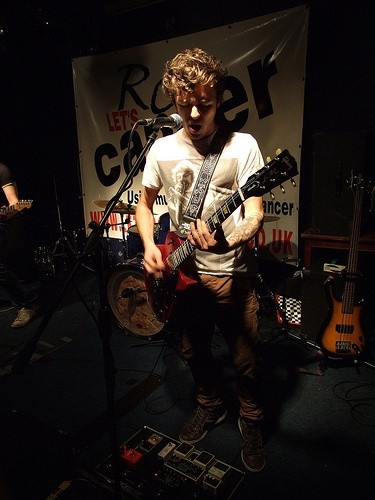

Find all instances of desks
(301, 227), (375, 270)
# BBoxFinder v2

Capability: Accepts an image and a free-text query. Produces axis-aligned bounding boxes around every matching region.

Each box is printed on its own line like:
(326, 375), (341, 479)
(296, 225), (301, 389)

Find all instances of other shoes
(11, 307), (36, 328)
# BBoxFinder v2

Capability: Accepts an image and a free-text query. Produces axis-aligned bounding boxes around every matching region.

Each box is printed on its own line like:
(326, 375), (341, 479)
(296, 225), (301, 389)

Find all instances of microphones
(122, 287), (135, 298)
(139, 113), (184, 127)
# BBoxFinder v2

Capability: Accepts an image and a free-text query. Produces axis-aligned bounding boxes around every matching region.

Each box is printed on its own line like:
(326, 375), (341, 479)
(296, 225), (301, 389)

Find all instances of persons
(0, 162), (40, 328)
(135, 48), (266, 472)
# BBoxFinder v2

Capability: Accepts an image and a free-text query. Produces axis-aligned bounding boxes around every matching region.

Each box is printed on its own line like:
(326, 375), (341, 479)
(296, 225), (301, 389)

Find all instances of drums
(102, 254), (176, 337)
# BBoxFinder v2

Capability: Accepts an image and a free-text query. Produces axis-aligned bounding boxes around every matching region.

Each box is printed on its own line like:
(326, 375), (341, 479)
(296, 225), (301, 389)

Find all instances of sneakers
(238, 417), (266, 472)
(179, 405), (227, 445)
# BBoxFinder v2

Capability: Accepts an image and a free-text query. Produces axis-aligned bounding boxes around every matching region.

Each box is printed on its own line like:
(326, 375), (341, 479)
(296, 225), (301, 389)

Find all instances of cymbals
(92, 200), (139, 214)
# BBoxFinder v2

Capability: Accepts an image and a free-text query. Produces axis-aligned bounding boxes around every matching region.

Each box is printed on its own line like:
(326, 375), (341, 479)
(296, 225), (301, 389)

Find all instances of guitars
(0, 199), (34, 219)
(145, 147), (300, 323)
(316, 183), (368, 366)
